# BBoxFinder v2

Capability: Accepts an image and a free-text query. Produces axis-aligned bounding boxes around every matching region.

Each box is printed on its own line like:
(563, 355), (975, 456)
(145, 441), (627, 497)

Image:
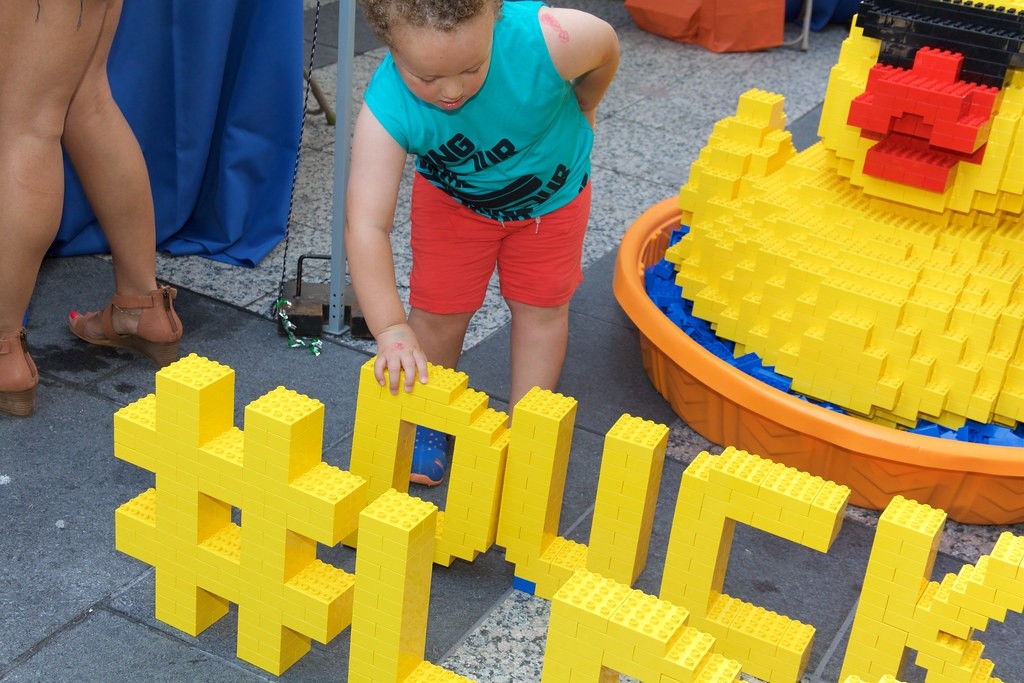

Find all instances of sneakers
(408, 425), (449, 486)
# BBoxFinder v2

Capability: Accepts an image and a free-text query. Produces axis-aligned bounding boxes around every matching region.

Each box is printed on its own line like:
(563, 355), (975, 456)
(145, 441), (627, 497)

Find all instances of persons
(1, 2), (185, 415)
(345, 0), (620, 488)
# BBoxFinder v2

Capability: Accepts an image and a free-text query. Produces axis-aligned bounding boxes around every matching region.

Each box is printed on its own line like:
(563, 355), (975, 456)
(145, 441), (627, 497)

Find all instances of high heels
(64, 283), (182, 369)
(0, 328), (38, 417)
